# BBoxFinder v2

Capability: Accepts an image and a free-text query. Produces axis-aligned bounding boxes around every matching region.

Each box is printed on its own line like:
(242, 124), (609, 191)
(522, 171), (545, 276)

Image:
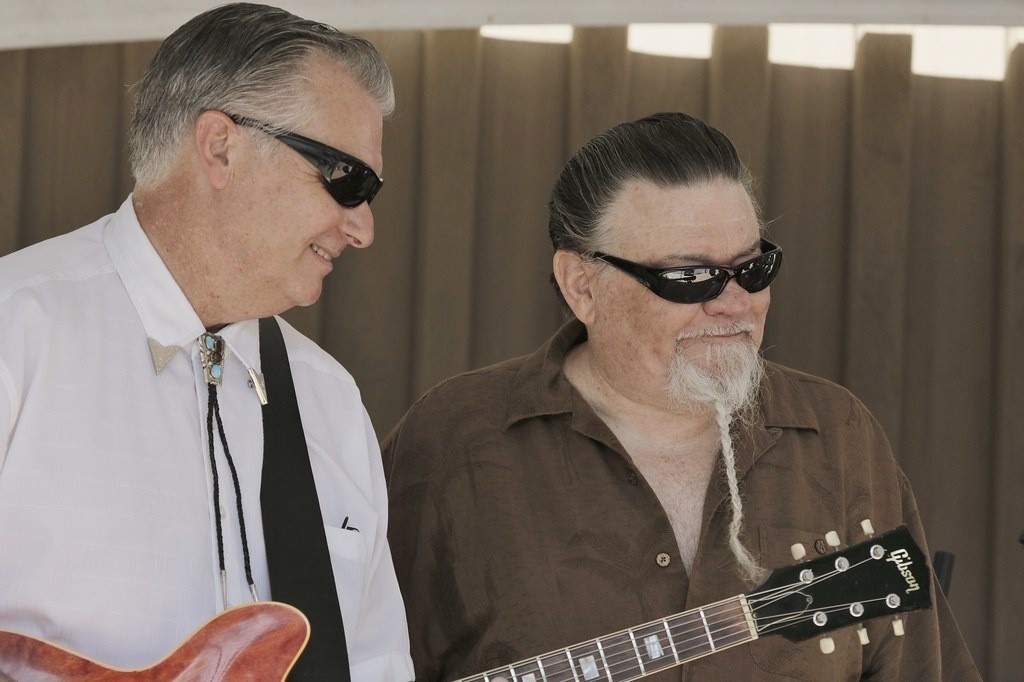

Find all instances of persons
(381, 111), (980, 682)
(0, 3), (415, 682)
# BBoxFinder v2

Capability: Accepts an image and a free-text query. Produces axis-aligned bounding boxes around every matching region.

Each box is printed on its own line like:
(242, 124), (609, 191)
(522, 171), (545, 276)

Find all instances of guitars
(0, 519), (935, 681)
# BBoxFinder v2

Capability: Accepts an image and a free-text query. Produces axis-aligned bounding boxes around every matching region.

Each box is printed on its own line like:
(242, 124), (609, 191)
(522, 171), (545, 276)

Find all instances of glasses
(198, 106), (384, 209)
(581, 236), (783, 304)
